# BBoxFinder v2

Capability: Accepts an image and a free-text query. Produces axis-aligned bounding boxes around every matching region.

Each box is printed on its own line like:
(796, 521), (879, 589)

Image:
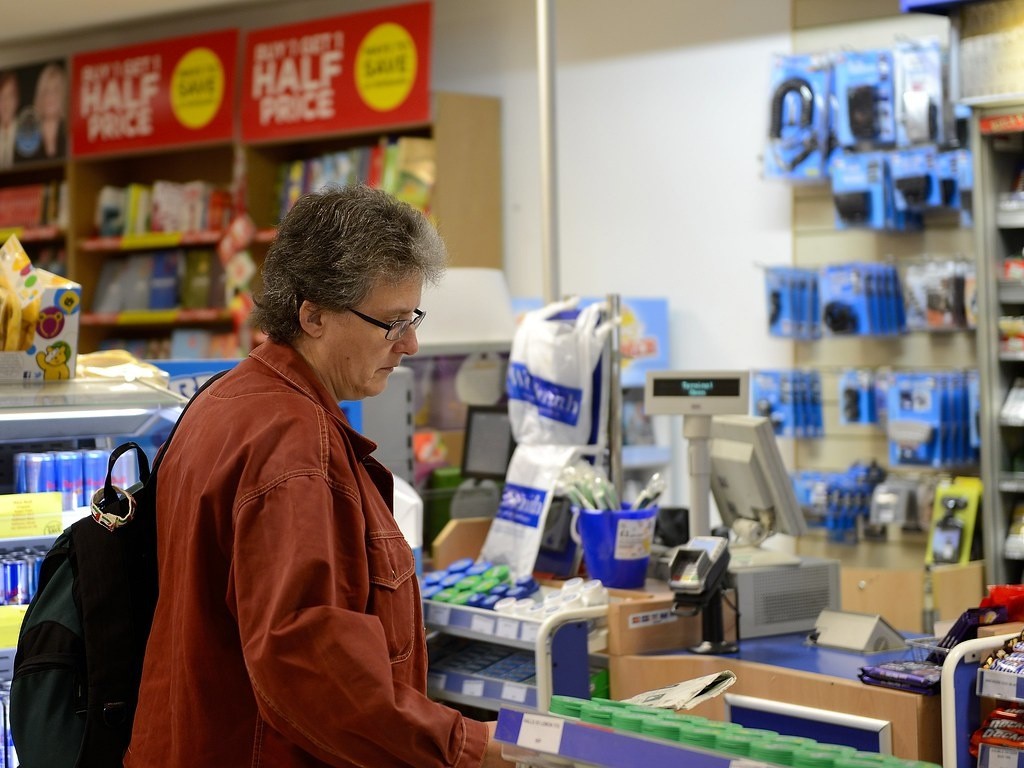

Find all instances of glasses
(349, 301), (427, 341)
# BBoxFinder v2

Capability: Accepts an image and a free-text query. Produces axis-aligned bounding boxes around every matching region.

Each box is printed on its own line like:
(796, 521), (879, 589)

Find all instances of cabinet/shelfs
(0, 87), (505, 355)
(962, 90), (1024, 598)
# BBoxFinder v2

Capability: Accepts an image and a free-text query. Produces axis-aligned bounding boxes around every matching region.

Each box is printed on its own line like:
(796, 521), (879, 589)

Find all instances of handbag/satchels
(506, 297), (619, 447)
(10, 441), (167, 768)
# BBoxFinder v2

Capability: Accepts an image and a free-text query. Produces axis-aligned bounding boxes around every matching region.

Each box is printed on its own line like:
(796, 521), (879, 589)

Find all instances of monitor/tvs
(707, 414), (808, 539)
(460, 405), (517, 482)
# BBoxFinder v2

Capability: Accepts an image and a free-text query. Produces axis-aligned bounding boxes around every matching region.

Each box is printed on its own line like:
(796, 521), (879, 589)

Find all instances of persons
(122, 185), (517, 768)
(14, 64), (66, 164)
(0, 72), (21, 168)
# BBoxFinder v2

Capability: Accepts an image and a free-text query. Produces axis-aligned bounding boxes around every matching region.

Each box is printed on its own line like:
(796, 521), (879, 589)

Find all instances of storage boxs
(0, 266), (81, 384)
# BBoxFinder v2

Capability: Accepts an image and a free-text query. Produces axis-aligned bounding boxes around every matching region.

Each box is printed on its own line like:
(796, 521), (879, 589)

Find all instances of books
(92, 179), (237, 360)
(273, 137), (433, 226)
